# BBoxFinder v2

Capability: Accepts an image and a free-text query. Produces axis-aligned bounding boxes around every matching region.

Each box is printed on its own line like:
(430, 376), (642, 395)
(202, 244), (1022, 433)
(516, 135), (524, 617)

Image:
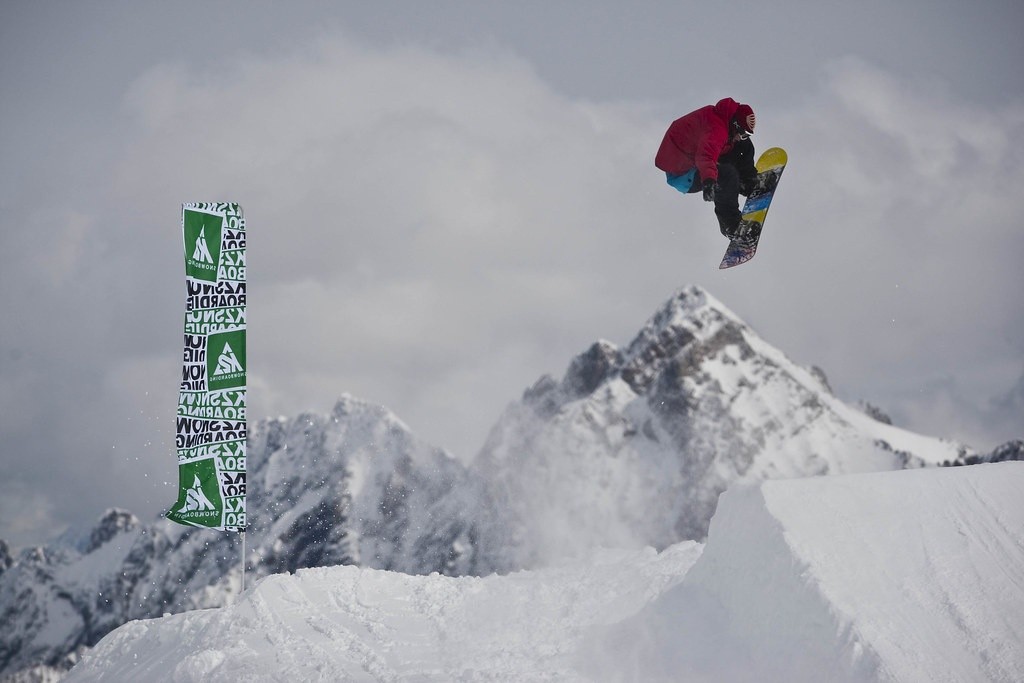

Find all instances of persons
(654, 98), (775, 244)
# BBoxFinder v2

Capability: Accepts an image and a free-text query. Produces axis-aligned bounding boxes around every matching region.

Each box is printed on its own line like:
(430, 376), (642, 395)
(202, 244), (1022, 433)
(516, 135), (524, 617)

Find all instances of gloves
(703, 178), (721, 201)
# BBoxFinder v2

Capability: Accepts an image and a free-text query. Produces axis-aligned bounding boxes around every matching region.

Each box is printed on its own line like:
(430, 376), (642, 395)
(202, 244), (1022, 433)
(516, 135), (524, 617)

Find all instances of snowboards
(718, 146), (788, 269)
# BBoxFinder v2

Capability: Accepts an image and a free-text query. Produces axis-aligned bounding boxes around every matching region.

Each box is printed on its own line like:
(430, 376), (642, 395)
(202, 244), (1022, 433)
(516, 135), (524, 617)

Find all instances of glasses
(734, 122), (752, 141)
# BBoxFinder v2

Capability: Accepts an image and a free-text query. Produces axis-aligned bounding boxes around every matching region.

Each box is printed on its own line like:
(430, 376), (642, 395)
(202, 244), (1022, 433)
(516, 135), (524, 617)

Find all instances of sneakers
(720, 219), (760, 242)
(745, 170), (777, 200)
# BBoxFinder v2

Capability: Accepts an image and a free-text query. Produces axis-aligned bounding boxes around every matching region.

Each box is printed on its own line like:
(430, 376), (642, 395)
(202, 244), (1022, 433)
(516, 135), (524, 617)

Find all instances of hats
(734, 103), (754, 134)
